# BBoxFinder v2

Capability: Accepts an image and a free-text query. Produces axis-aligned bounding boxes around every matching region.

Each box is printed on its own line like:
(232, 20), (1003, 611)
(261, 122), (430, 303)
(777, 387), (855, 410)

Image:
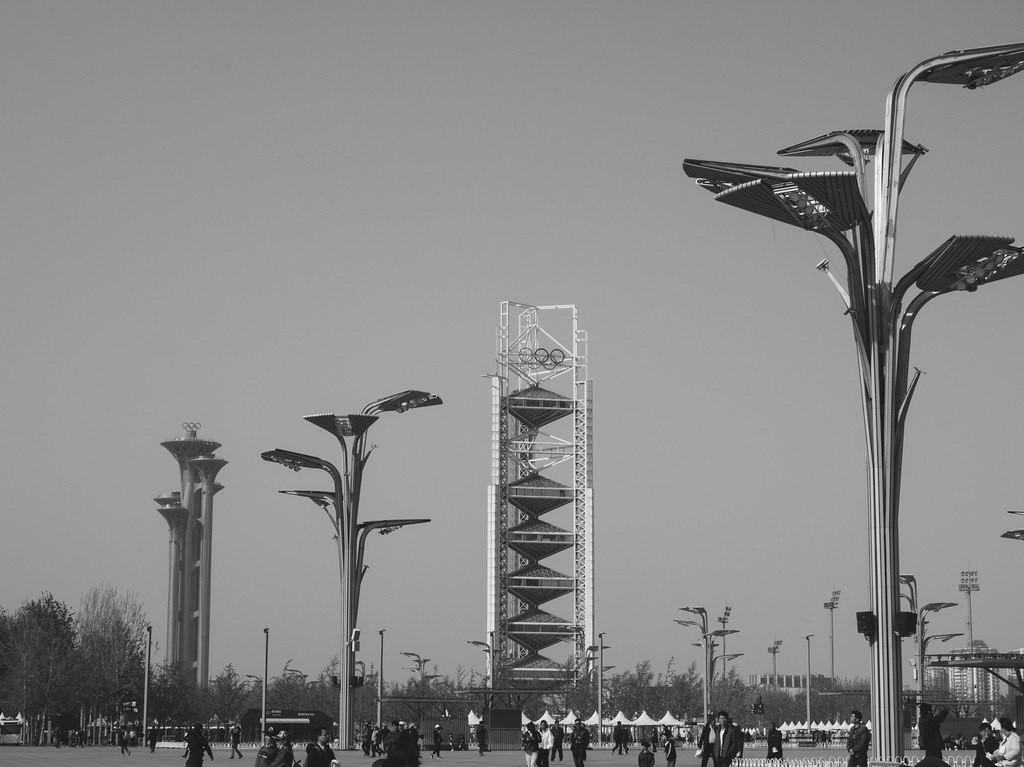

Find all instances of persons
(638, 724), (676, 767)
(203, 724), (214, 761)
(746, 731), (751, 742)
(752, 730), (764, 742)
(355, 724), (390, 757)
(846, 703), (1022, 767)
(697, 712), (745, 767)
(570, 718), (590, 767)
(384, 720), (443, 759)
(372, 735), (420, 767)
(766, 723), (783, 762)
(181, 724), (192, 758)
(255, 726), (339, 767)
(611, 720), (633, 755)
(522, 720), (564, 767)
(120, 725), (157, 755)
(185, 723), (213, 767)
(783, 729), (968, 751)
(229, 726), (243, 759)
(476, 721), (487, 756)
(448, 733), (465, 751)
(681, 730), (693, 745)
(53, 726), (85, 749)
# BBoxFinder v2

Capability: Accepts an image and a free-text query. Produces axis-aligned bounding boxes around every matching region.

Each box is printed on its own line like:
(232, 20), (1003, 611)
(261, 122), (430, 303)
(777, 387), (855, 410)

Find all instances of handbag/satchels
(694, 749), (704, 761)
(275, 749), (302, 767)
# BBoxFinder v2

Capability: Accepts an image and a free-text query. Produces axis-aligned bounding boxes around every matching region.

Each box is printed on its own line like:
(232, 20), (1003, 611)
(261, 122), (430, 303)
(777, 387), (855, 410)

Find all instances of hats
(435, 724), (440, 729)
(399, 721), (405, 725)
(264, 727), (280, 737)
(273, 731), (289, 741)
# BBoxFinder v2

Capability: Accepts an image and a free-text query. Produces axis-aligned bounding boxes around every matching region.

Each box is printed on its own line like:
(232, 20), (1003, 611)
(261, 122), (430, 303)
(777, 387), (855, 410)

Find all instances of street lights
(260, 626), (270, 749)
(799, 634), (816, 740)
(677, 40), (1024, 767)
(260, 390), (444, 751)
(594, 631), (607, 747)
(822, 585), (843, 687)
(377, 626), (388, 730)
(672, 605), (741, 729)
(717, 604), (731, 683)
(141, 624), (154, 749)
(768, 638), (783, 691)
(958, 568), (981, 657)
(898, 573), (968, 749)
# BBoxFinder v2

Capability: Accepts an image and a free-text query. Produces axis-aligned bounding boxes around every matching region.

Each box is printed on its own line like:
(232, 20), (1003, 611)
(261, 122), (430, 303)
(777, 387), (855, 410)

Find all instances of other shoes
(437, 757), (443, 760)
(430, 755), (433, 760)
(238, 755), (243, 760)
(229, 756), (234, 759)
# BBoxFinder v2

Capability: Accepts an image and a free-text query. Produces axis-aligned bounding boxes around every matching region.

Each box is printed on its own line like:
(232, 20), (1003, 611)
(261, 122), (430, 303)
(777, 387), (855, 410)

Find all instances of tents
(776, 720), (872, 730)
(584, 710), (685, 745)
(468, 709), (584, 733)
